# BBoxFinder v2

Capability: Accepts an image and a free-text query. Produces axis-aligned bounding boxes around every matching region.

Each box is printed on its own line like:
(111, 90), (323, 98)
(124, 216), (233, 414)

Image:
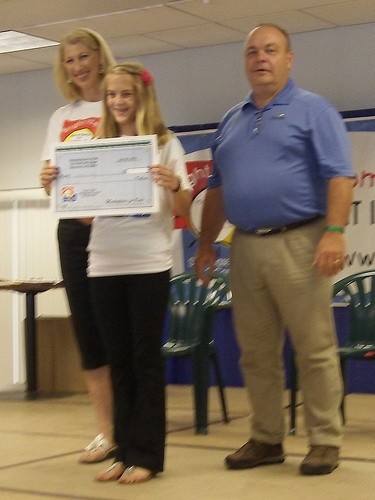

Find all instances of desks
(1, 279), (70, 400)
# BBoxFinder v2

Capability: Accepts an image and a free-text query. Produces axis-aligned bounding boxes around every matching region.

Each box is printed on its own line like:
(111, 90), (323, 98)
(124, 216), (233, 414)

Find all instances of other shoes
(79, 433), (119, 464)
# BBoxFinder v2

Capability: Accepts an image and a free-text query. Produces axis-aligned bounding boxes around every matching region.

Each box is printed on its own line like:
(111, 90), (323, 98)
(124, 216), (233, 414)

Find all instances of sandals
(97, 461), (157, 484)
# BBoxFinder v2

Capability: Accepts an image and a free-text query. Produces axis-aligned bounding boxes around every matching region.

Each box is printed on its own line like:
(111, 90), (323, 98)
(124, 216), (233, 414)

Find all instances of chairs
(290, 271), (375, 433)
(160, 270), (231, 435)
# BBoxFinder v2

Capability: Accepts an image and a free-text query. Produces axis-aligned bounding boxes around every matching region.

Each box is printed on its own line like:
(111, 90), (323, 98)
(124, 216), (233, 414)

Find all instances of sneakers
(299, 445), (341, 474)
(225, 439), (285, 469)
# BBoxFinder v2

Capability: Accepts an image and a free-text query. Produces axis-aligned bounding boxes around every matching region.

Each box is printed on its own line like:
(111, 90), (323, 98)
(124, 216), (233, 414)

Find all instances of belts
(255, 214), (324, 237)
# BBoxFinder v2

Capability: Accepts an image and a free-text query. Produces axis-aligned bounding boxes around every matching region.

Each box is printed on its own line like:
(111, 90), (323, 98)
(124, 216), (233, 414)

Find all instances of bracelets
(325, 225), (346, 233)
(172, 181), (181, 192)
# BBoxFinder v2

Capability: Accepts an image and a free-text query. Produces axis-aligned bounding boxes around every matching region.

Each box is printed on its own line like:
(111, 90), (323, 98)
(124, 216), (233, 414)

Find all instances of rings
(334, 258), (342, 265)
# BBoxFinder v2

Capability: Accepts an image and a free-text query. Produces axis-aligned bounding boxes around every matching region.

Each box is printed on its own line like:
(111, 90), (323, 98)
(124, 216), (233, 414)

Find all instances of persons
(40, 27), (194, 485)
(194, 22), (358, 475)
